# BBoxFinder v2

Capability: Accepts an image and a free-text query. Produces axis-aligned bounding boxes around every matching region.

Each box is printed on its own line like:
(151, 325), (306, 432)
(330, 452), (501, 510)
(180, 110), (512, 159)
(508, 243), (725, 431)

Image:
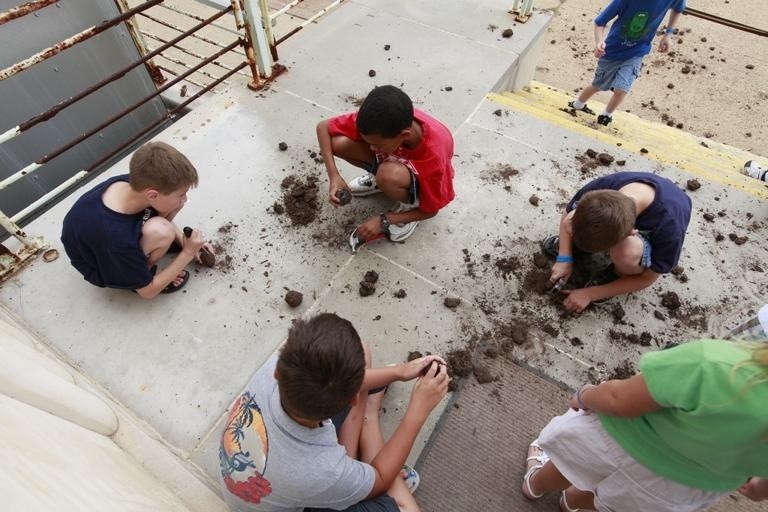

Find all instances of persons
(59, 141), (214, 299)
(521, 303), (768, 511)
(219, 314), (453, 511)
(539, 170), (693, 313)
(315, 85), (455, 243)
(566, 0), (687, 126)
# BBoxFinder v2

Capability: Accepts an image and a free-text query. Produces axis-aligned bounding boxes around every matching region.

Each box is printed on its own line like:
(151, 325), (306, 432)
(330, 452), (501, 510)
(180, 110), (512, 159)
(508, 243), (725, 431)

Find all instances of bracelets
(665, 28), (674, 34)
(576, 383), (595, 410)
(380, 212), (390, 232)
(554, 255), (574, 263)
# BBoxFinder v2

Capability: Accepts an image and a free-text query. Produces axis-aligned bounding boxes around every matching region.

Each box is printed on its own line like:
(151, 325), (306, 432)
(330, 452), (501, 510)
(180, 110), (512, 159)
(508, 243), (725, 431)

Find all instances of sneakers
(585, 262), (619, 304)
(347, 172), (379, 194)
(388, 203), (416, 242)
(742, 160), (768, 181)
(568, 101), (596, 116)
(597, 114), (611, 125)
(542, 234), (589, 260)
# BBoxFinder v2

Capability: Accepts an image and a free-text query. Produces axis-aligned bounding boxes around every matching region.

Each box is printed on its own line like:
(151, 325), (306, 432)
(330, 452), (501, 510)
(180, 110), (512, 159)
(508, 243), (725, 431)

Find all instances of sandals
(523, 438), (551, 498)
(131, 265), (189, 293)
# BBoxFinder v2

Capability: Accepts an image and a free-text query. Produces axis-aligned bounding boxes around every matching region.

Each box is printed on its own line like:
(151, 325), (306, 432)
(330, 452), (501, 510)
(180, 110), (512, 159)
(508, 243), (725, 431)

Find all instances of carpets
(413, 337), (768, 512)
